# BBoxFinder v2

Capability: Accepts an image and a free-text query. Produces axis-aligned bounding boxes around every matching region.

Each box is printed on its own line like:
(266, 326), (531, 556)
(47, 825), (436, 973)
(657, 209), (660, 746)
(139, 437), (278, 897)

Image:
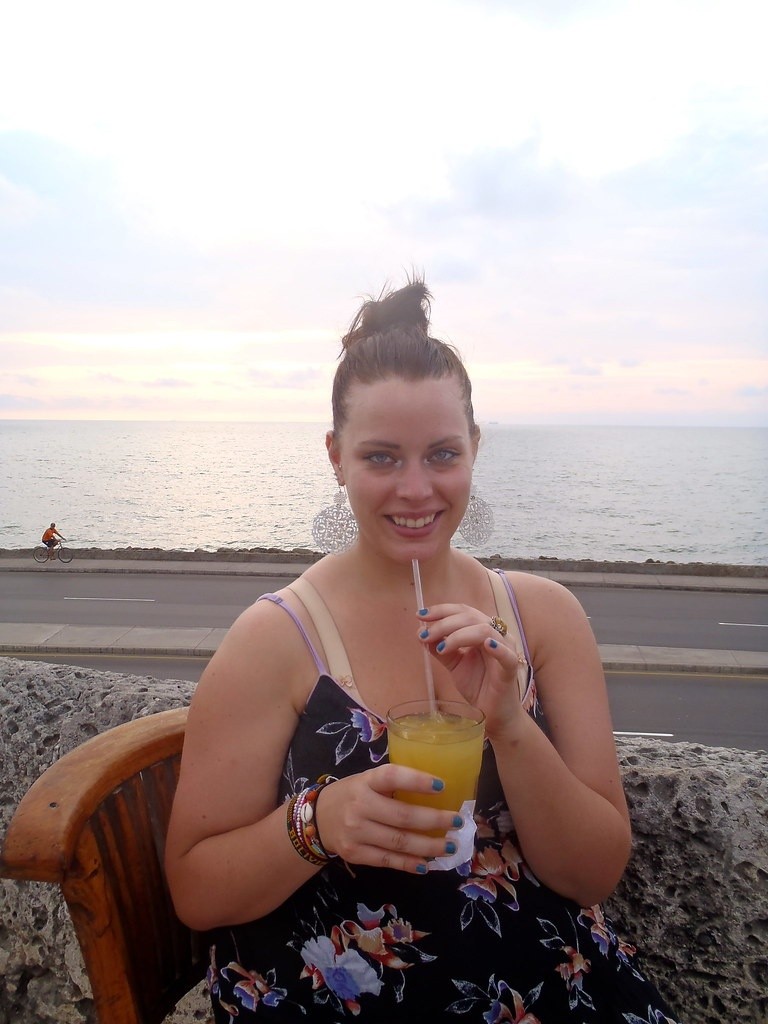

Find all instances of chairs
(0, 705), (208, 1024)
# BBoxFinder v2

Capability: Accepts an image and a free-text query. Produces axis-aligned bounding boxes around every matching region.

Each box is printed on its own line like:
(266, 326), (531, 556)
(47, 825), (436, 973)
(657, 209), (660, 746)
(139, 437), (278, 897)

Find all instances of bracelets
(285, 772), (343, 867)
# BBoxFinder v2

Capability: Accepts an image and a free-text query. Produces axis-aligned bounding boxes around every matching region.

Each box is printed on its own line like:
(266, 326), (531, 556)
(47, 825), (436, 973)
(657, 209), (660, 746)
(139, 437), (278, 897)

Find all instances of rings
(489, 614), (509, 637)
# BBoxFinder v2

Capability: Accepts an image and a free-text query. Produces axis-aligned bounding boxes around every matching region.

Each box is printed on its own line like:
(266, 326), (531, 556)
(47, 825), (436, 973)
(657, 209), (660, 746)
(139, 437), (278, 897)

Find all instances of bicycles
(34, 540), (74, 563)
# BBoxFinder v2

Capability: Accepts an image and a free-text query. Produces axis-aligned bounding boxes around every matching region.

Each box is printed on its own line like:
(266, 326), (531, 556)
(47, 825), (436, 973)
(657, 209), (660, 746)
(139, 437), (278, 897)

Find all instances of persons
(42, 522), (66, 560)
(159, 265), (683, 1024)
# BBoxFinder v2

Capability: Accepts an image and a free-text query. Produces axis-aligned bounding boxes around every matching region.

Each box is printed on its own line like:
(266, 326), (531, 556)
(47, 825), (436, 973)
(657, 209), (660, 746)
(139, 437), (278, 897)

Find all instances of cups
(386, 699), (486, 839)
